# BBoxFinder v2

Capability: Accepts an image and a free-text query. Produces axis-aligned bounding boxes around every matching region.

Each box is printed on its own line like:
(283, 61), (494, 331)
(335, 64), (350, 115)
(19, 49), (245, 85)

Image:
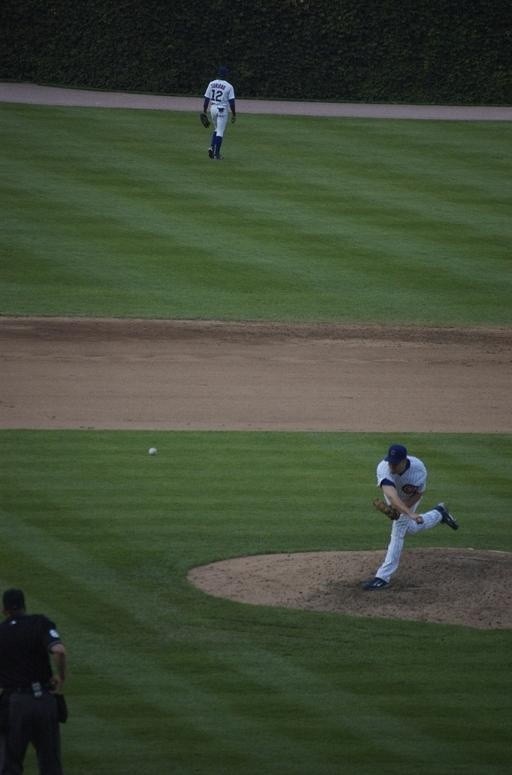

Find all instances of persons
(204, 66), (236, 161)
(0, 589), (66, 775)
(362, 444), (459, 591)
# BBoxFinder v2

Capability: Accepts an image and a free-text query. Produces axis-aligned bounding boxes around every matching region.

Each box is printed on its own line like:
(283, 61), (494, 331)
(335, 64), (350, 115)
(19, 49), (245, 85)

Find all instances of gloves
(231, 116), (236, 124)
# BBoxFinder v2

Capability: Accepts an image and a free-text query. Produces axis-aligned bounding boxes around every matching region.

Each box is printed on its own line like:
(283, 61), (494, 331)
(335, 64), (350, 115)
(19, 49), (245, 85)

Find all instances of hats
(2, 588), (26, 611)
(384, 444), (407, 465)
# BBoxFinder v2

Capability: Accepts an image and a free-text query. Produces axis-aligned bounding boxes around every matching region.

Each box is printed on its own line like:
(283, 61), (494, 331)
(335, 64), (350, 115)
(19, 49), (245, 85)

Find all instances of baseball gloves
(200, 112), (209, 128)
(374, 500), (400, 520)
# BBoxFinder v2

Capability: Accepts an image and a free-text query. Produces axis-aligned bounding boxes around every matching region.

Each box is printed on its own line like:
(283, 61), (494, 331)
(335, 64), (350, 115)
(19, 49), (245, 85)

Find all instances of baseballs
(149, 448), (157, 456)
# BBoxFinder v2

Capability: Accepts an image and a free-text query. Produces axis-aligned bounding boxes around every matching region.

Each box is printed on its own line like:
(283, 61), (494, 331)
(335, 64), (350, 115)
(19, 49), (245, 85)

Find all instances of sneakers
(208, 147), (215, 160)
(214, 155), (224, 160)
(433, 502), (460, 532)
(361, 577), (391, 591)
